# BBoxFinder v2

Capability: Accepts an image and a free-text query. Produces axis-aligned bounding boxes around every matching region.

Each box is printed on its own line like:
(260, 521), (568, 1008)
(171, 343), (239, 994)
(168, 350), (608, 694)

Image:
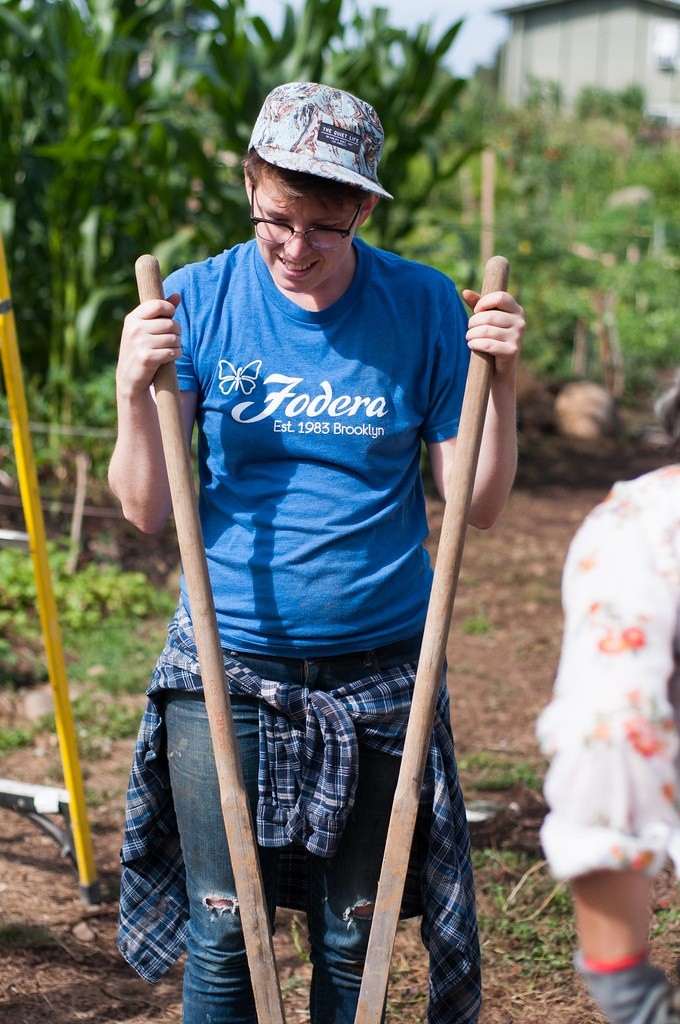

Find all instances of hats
(247, 80), (395, 201)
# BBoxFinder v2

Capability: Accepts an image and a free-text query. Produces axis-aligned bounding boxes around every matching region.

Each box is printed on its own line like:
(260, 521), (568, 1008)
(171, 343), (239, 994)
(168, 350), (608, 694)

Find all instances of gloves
(573, 948), (680, 1024)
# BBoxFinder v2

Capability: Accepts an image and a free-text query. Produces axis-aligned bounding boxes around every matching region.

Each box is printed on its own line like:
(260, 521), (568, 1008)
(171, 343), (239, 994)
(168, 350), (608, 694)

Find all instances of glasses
(250, 181), (362, 251)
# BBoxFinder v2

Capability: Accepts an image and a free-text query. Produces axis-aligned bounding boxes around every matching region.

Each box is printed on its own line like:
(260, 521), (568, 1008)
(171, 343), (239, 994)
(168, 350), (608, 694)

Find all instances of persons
(108, 80), (527, 1023)
(536, 366), (680, 1023)
